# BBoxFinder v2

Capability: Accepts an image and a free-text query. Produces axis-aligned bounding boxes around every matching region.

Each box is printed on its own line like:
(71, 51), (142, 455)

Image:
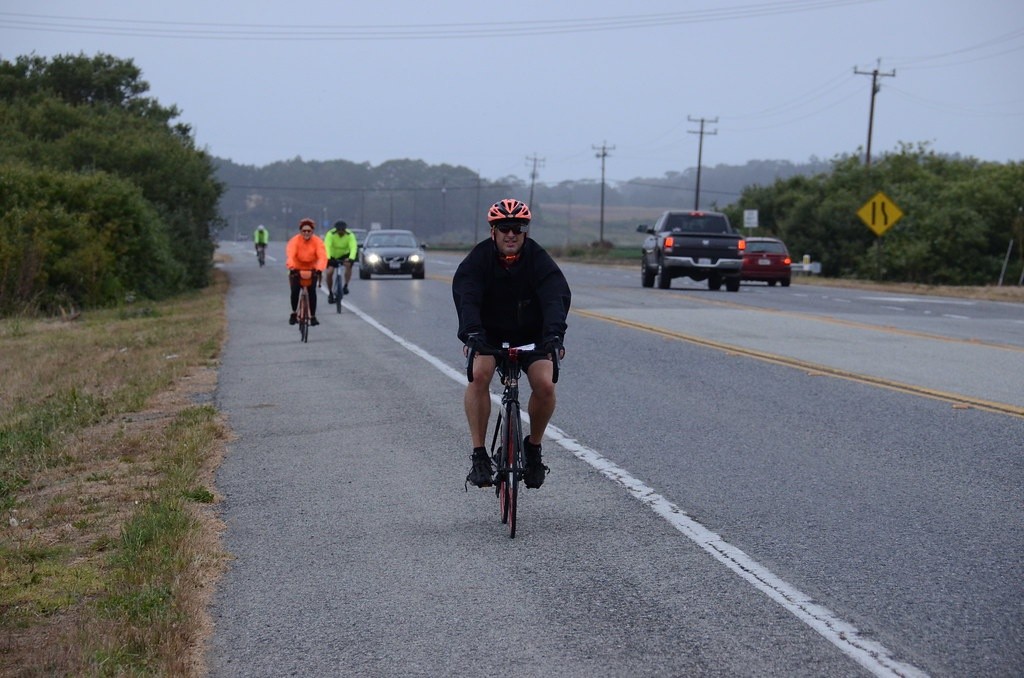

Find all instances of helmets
(299, 218), (315, 231)
(334, 220), (347, 229)
(487, 198), (531, 231)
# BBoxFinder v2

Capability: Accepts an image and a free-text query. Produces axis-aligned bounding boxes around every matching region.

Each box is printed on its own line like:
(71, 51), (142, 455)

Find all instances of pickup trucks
(635, 209), (745, 292)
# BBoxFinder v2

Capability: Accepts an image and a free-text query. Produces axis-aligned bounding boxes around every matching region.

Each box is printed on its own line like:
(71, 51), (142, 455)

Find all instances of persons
(324, 221), (357, 304)
(452, 197), (573, 489)
(254, 224), (269, 264)
(285, 218), (328, 326)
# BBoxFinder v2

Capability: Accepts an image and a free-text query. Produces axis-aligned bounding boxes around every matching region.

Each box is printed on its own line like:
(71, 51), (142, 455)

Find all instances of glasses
(337, 228), (345, 231)
(301, 229), (312, 233)
(494, 224), (529, 234)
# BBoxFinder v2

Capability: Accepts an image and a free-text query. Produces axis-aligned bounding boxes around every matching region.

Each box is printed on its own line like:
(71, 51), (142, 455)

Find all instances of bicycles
(325, 256), (358, 313)
(465, 332), (563, 539)
(289, 268), (322, 343)
(255, 242), (268, 268)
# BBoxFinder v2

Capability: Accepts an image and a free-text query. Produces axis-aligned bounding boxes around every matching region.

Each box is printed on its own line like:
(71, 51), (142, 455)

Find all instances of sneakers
(469, 447), (492, 486)
(341, 286), (349, 294)
(328, 294), (335, 304)
(310, 317), (320, 325)
(523, 435), (544, 488)
(289, 313), (298, 324)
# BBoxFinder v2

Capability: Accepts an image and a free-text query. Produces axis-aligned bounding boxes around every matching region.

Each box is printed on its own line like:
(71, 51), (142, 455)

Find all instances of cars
(350, 228), (368, 261)
(358, 230), (427, 279)
(738, 236), (791, 286)
